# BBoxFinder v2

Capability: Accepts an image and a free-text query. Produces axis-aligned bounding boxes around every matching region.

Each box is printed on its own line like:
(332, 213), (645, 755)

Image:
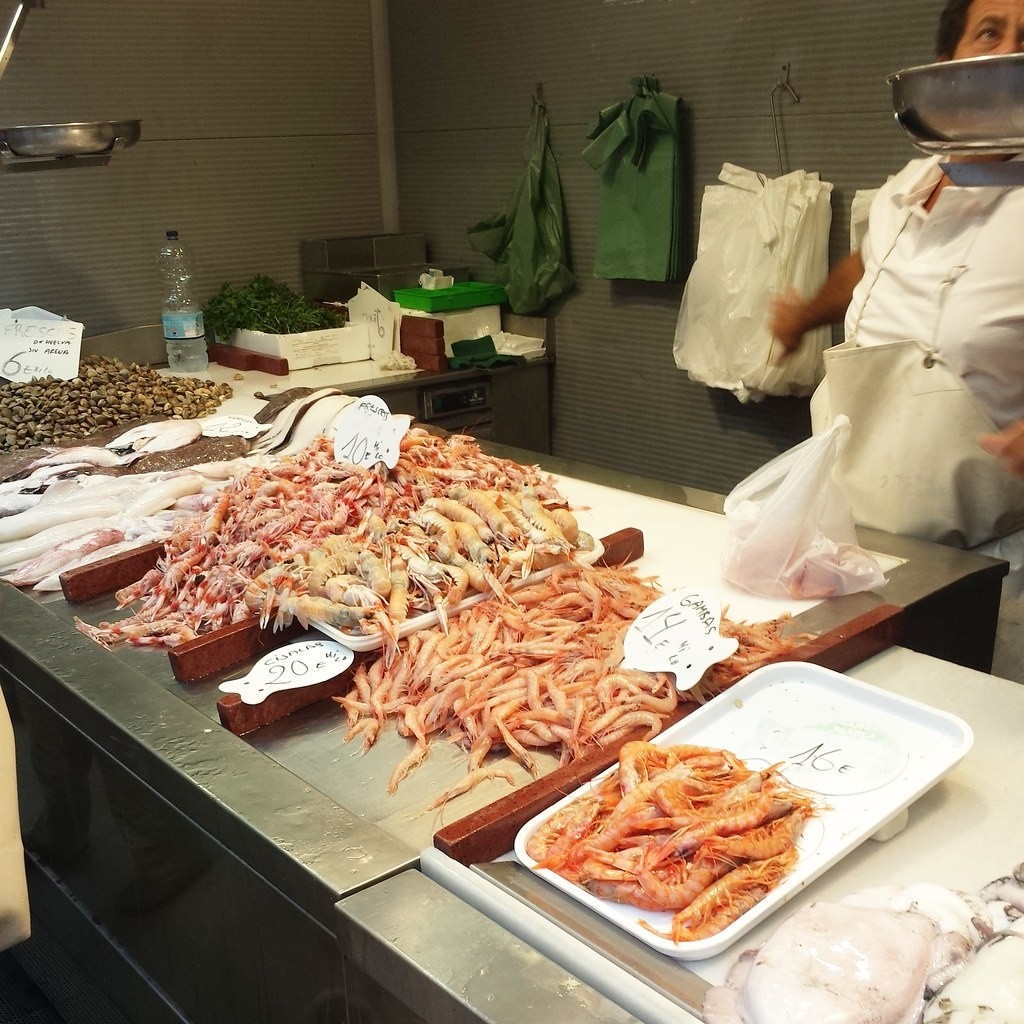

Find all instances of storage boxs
(396, 304), (502, 358)
(212, 318), (372, 371)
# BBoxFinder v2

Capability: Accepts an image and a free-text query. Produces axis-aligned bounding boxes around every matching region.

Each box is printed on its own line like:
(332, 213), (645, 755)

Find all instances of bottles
(155, 230), (208, 372)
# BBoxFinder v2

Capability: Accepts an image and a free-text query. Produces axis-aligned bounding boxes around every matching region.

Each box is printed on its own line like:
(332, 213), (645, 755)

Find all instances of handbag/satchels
(466, 100), (580, 316)
(720, 413), (891, 602)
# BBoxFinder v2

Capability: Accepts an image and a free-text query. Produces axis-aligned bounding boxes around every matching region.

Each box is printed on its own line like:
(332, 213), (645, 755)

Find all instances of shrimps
(72, 428), (838, 837)
(525, 741), (836, 945)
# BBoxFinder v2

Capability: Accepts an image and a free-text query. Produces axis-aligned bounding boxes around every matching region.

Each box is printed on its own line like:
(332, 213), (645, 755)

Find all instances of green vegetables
(201, 274), (346, 342)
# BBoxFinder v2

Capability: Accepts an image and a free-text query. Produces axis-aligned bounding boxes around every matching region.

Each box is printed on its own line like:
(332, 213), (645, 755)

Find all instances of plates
(514, 660), (974, 963)
(306, 528), (604, 651)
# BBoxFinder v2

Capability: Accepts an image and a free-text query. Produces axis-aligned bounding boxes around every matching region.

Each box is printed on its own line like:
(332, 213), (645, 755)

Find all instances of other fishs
(888, 864), (1024, 1022)
(701, 901), (940, 1024)
(0, 386), (357, 592)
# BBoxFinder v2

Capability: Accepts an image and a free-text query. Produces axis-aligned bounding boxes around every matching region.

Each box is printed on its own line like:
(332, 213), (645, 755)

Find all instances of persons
(772, 0), (1024, 676)
